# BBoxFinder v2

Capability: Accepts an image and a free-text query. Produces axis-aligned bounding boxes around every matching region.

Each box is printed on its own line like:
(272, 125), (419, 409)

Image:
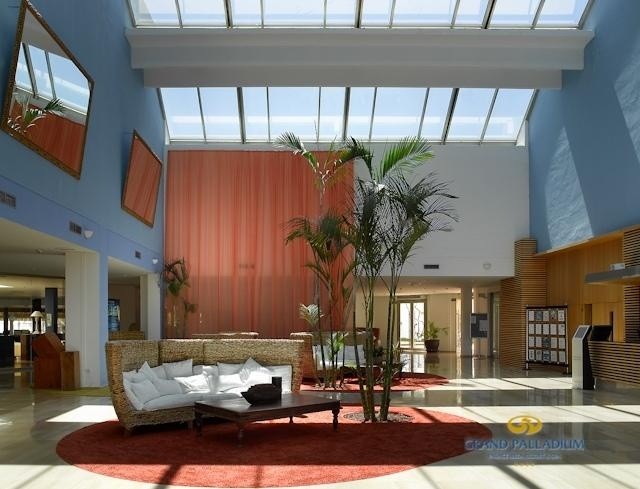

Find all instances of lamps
(30, 310), (43, 335)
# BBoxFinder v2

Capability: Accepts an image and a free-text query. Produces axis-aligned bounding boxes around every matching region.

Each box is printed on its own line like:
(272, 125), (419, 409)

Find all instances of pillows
(313, 345), (364, 361)
(126, 360), (279, 403)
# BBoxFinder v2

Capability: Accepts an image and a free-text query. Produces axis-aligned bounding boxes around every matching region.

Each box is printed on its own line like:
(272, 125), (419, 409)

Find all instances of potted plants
(424, 321), (450, 352)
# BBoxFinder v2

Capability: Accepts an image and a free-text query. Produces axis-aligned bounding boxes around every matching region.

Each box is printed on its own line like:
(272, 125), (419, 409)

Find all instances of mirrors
(0, 1), (94, 180)
(121, 130), (164, 228)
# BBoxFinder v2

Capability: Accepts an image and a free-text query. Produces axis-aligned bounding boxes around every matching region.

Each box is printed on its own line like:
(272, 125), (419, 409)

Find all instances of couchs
(290, 331), (373, 384)
(106, 338), (305, 435)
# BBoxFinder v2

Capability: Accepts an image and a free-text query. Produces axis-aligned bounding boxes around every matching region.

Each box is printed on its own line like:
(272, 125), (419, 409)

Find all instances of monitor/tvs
(574, 326), (589, 339)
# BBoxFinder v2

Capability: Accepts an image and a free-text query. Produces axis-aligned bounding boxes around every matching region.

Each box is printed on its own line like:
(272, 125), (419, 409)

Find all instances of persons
(362, 336), (382, 365)
(374, 340), (383, 364)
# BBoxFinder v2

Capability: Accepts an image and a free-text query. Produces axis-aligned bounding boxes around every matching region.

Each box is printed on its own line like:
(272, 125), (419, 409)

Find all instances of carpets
(300, 372), (448, 393)
(56, 403), (492, 489)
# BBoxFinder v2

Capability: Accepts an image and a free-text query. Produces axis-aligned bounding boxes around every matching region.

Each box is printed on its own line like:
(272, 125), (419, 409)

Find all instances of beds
(193, 393), (342, 446)
